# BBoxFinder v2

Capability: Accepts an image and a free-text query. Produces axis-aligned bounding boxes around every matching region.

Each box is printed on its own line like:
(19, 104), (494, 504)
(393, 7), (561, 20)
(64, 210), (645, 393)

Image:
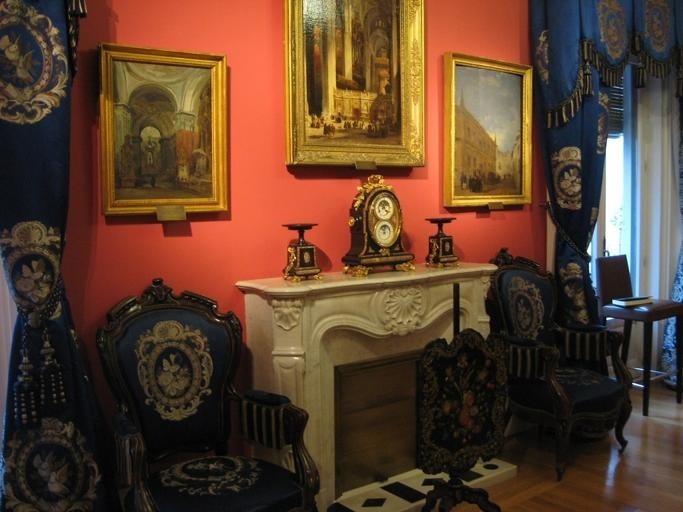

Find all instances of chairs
(97, 276), (320, 512)
(485, 259), (635, 482)
(594, 255), (683, 417)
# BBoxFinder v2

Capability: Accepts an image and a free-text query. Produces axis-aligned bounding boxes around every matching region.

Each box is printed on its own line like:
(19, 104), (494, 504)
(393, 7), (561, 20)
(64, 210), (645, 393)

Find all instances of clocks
(342, 173), (415, 277)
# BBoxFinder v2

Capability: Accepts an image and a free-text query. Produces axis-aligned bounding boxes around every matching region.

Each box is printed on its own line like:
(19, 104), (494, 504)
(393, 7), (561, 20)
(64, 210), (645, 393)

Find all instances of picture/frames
(440, 51), (534, 211)
(99, 42), (231, 220)
(283, 0), (426, 172)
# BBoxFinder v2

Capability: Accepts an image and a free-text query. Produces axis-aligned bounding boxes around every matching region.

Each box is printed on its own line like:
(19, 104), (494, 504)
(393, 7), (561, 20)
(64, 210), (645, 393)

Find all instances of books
(611, 295), (653, 308)
